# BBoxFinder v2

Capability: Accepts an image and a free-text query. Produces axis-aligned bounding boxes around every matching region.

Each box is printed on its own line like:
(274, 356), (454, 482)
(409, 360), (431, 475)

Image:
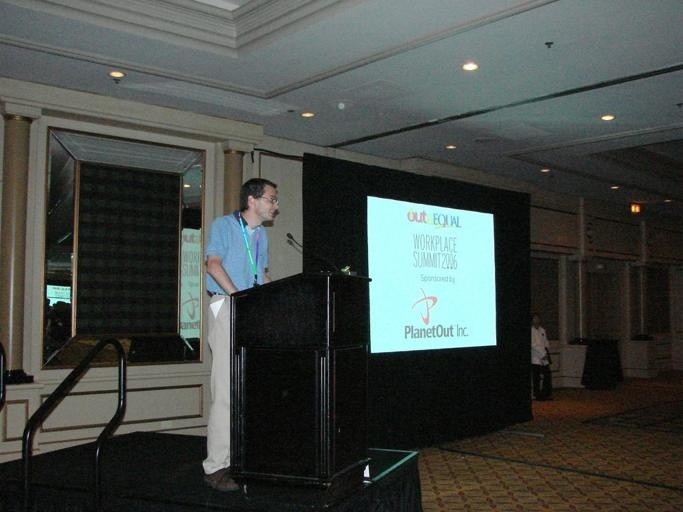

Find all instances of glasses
(257, 194), (279, 205)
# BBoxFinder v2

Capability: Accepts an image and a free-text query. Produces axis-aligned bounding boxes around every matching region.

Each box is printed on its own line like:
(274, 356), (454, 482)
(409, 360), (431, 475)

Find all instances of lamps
(630, 203), (641, 214)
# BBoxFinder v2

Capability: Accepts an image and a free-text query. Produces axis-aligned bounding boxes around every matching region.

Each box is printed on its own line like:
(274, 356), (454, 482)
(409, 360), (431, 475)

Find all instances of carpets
(580, 401), (683, 434)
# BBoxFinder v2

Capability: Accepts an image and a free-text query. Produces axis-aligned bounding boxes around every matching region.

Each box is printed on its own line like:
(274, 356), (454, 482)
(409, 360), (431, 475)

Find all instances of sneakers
(206, 474), (240, 492)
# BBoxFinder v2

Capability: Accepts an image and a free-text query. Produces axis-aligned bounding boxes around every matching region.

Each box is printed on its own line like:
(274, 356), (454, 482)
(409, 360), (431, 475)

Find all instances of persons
(531, 312), (554, 402)
(201, 177), (280, 492)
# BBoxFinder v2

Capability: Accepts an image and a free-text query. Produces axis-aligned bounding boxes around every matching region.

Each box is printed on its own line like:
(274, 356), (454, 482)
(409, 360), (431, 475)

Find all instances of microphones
(287, 233), (344, 275)
